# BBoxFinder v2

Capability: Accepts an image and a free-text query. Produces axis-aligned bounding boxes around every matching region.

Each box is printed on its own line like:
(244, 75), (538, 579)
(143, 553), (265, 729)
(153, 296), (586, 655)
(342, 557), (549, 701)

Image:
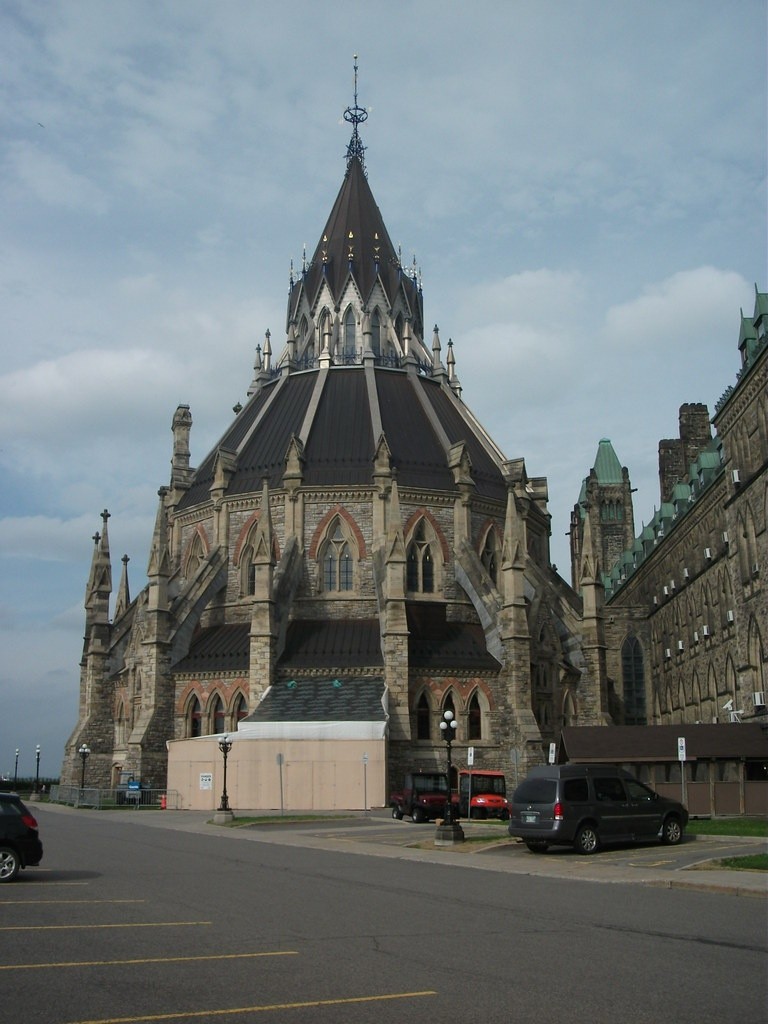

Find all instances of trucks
(388, 769), (510, 822)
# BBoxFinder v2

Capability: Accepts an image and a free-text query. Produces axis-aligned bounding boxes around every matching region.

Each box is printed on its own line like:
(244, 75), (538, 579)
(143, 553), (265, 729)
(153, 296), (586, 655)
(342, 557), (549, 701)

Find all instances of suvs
(0, 792), (44, 884)
(508, 760), (690, 856)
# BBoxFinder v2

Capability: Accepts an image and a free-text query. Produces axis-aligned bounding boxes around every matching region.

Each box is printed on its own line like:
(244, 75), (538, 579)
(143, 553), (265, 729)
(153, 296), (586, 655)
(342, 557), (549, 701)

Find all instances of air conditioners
(712, 717), (718, 724)
(683, 568), (689, 578)
(633, 564), (636, 568)
(752, 691), (765, 707)
(618, 580), (621, 584)
(664, 586), (669, 594)
(727, 610), (734, 621)
(678, 641), (684, 650)
(752, 563), (758, 573)
(721, 532), (729, 543)
(670, 579), (676, 589)
(653, 595), (658, 604)
(657, 531), (664, 537)
(704, 548), (711, 559)
(693, 631), (698, 641)
(622, 574), (626, 580)
(702, 625), (708, 636)
(731, 469), (740, 484)
(665, 649), (671, 658)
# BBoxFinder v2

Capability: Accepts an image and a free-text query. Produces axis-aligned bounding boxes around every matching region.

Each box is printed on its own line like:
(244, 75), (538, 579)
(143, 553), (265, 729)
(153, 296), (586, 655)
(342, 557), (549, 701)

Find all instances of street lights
(216, 731), (233, 811)
(34, 744), (42, 793)
(13, 748), (20, 792)
(439, 709), (460, 827)
(78, 743), (91, 797)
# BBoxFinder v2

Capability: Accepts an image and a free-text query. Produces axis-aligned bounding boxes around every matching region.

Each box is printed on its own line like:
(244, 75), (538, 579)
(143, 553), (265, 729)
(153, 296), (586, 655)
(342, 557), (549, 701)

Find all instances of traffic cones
(160, 794), (168, 812)
